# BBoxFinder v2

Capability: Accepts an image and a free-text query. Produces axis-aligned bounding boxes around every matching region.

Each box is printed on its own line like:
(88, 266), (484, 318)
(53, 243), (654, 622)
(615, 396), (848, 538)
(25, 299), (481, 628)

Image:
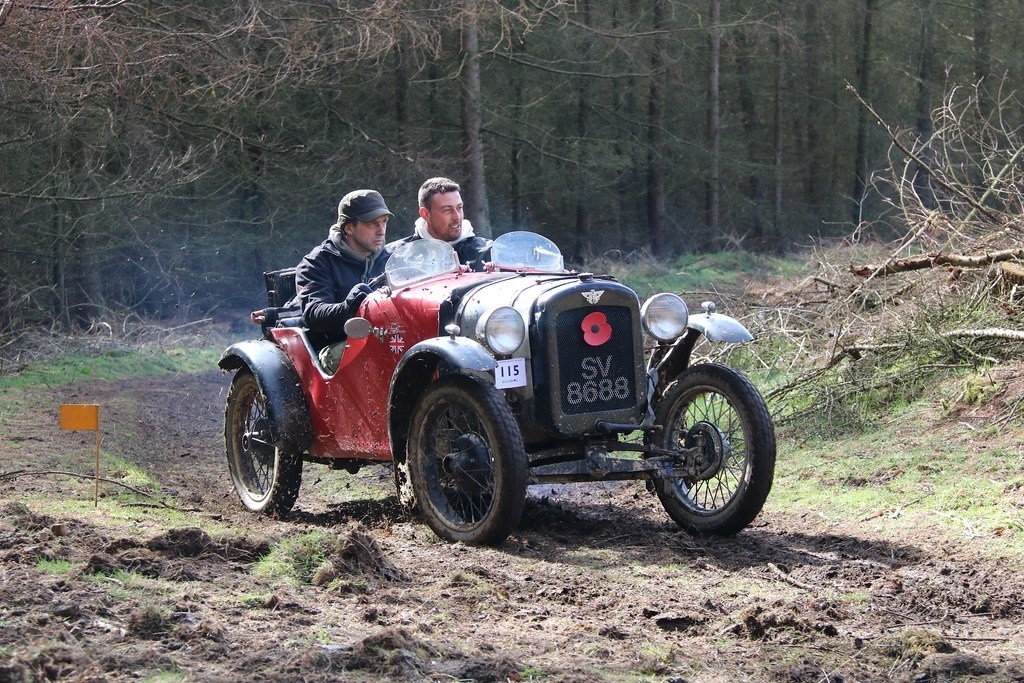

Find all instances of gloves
(345, 282), (373, 312)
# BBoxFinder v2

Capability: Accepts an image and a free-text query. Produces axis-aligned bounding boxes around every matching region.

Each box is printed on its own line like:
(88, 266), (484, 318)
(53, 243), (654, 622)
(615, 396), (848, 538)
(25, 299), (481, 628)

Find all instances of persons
(386, 176), (515, 287)
(297, 190), (425, 372)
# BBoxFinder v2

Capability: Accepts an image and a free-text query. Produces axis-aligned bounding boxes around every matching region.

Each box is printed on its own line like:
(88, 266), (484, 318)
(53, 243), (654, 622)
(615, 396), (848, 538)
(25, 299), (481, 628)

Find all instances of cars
(217, 242), (778, 553)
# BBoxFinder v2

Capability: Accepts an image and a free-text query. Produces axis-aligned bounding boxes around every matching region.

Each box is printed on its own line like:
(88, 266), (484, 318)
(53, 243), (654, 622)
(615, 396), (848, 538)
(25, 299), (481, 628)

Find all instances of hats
(337, 188), (396, 223)
